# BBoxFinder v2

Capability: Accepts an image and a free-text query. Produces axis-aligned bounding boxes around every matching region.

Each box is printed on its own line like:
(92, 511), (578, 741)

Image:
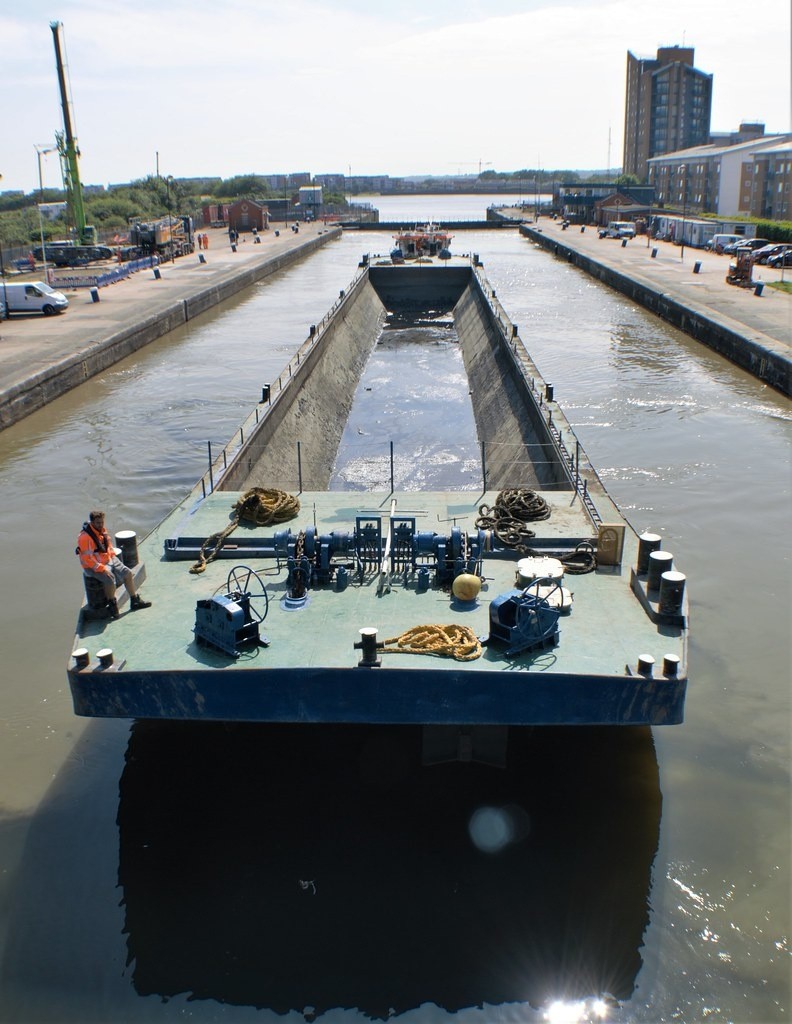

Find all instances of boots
(108, 598), (119, 617)
(131, 594), (151, 609)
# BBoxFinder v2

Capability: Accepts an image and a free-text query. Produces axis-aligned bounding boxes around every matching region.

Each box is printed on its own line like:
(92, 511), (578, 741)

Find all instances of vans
(0, 281), (70, 316)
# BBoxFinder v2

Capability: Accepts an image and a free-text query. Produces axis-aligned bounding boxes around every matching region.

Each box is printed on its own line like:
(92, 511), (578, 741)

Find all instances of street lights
(166, 175), (174, 264)
(27, 140), (49, 285)
(681, 163), (687, 258)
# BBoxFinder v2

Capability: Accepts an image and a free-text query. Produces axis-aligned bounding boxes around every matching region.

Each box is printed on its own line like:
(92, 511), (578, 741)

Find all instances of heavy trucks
(119, 213), (196, 263)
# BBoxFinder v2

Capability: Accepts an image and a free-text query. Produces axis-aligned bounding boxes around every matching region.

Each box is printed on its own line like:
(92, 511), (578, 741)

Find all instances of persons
(28, 251), (34, 266)
(197, 233), (208, 249)
(77, 511), (151, 619)
(117, 247), (121, 265)
(229, 229), (239, 246)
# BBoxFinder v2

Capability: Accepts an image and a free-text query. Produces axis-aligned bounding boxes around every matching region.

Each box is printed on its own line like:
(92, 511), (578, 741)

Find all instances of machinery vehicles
(32, 18), (114, 267)
(725, 246), (759, 289)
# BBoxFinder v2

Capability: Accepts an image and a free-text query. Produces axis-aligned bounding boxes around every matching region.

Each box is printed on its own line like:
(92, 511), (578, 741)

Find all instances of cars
(607, 221), (635, 239)
(705, 233), (792, 269)
